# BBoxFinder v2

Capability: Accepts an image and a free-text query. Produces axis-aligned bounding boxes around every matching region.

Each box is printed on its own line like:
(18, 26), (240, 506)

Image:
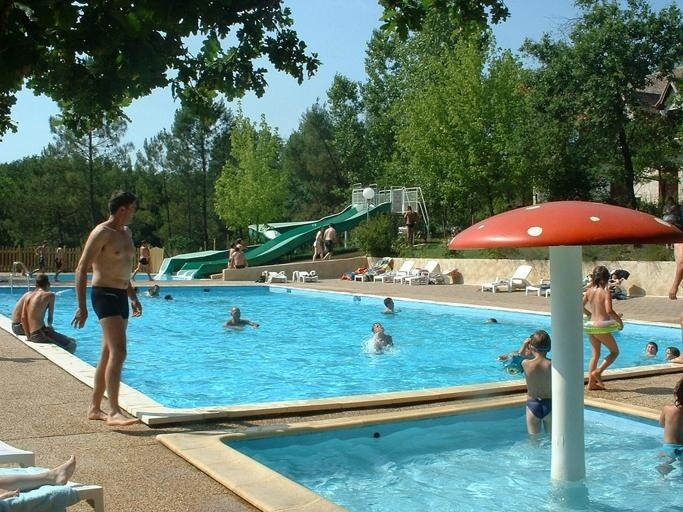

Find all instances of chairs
(405, 267), (457, 285)
(582, 274), (623, 298)
(374, 261), (415, 283)
(394, 261), (439, 285)
(526, 279), (551, 296)
(546, 273), (595, 298)
(354, 257), (393, 282)
(0, 439), (35, 469)
(292, 270), (319, 282)
(482, 265), (532, 293)
(262, 271), (288, 283)
(0, 466), (106, 512)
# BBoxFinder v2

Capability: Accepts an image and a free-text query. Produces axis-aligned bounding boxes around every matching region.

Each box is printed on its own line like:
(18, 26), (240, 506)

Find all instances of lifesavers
(583, 315), (619, 334)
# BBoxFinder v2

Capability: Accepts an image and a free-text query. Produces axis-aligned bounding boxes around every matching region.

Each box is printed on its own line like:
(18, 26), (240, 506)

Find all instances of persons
(383, 298), (397, 314)
(55, 242), (65, 281)
(12, 282), (50, 335)
(36, 240), (48, 273)
(372, 322), (392, 346)
(228, 238), (248, 270)
(0, 455), (75, 499)
(521, 331), (551, 435)
(223, 307), (259, 330)
(669, 243), (683, 299)
(146, 285), (159, 297)
(659, 379), (683, 462)
(21, 275), (76, 354)
(583, 265), (624, 390)
(519, 334), (534, 356)
(13, 262), (27, 276)
(666, 347), (683, 363)
(312, 227), (324, 262)
(404, 206), (414, 246)
(322, 223), (336, 260)
(645, 342), (658, 358)
(71, 191), (143, 426)
(131, 241), (154, 282)
(371, 333), (386, 355)
(663, 196), (677, 248)
